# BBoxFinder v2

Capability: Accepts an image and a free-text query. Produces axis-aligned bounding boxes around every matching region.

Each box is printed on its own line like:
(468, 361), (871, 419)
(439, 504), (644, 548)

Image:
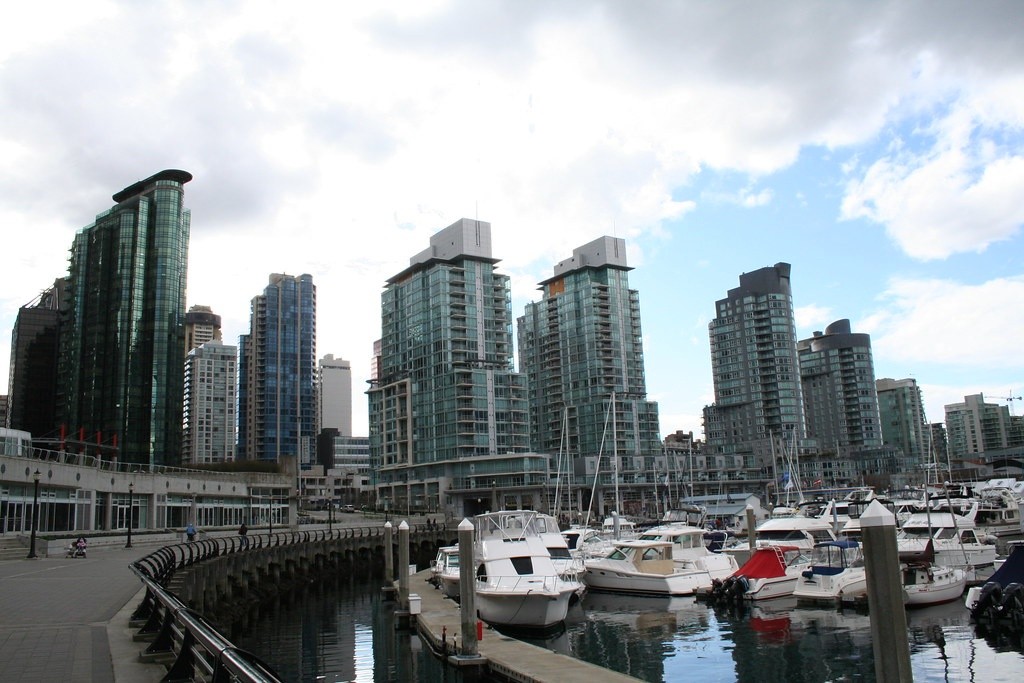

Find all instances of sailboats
(899, 378), (972, 604)
(768, 427), (826, 524)
(643, 433), (732, 552)
(549, 390), (635, 564)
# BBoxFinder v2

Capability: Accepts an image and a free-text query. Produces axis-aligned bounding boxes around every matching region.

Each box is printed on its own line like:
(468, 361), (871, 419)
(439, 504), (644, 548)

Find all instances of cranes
(984, 389), (1022, 416)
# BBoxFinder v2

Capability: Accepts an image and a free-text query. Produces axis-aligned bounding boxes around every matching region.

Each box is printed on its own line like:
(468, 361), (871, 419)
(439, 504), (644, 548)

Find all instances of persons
(70, 538), (87, 556)
(186, 524), (196, 541)
(238, 524), (247, 535)
(716, 518), (721, 530)
(427, 518), (430, 529)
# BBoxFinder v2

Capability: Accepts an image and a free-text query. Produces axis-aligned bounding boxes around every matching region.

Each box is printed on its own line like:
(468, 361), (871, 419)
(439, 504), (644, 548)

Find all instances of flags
(784, 480), (793, 490)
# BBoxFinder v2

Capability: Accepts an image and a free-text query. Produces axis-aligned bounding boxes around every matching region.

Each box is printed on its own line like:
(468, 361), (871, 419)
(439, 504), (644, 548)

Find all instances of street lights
(328, 493), (332, 533)
(125, 481), (133, 548)
(27, 467), (42, 558)
(268, 493), (273, 534)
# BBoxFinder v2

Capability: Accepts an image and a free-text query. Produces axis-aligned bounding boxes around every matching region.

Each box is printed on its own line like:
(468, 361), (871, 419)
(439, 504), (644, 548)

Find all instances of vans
(341, 505), (354, 512)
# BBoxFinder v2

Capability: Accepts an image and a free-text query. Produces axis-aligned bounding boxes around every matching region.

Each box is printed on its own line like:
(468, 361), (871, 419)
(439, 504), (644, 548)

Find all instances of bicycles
(186, 534), (195, 547)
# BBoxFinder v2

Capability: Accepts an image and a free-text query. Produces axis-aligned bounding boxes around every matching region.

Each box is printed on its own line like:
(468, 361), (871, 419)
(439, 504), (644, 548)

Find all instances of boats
(964, 540), (1024, 633)
(793, 539), (865, 602)
(641, 526), (740, 584)
(585, 539), (710, 596)
(472, 508), (586, 627)
(754, 470), (1024, 566)
(430, 543), (461, 597)
(708, 543), (813, 599)
